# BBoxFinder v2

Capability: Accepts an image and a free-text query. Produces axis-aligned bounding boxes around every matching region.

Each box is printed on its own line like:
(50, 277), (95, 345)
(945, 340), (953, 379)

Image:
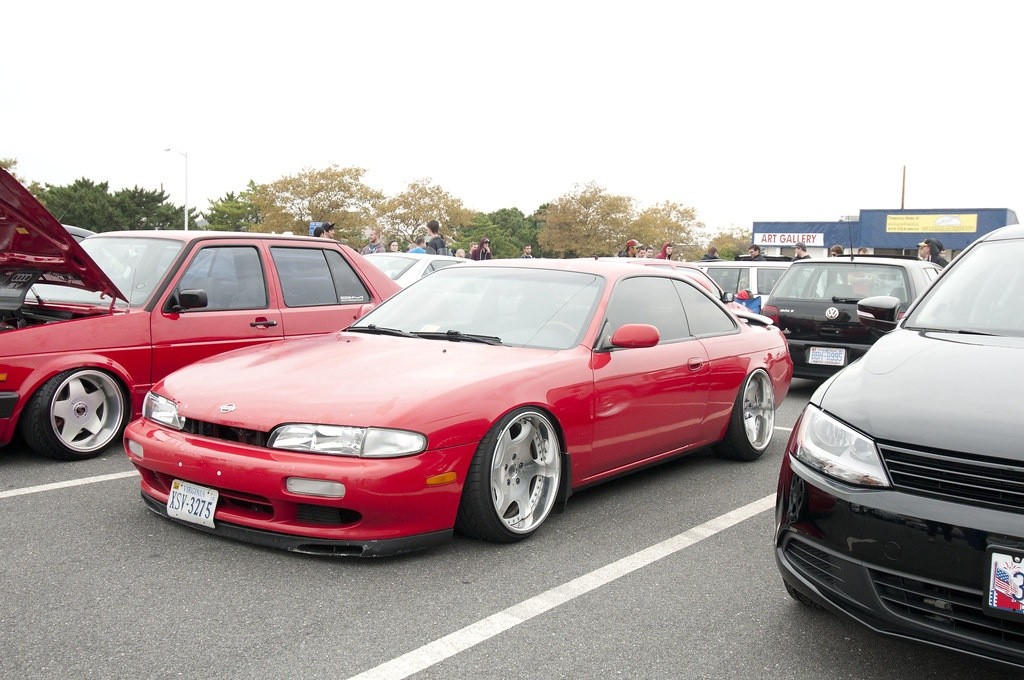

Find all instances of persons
(314, 221), (336, 239)
(617, 239), (654, 258)
(520, 243), (532, 258)
(406, 238), (426, 253)
(747, 244), (766, 261)
(793, 242), (811, 260)
(388, 239), (398, 252)
(830, 245), (844, 257)
(655, 242), (673, 260)
(455, 237), (491, 261)
(425, 219), (446, 255)
(701, 247), (719, 260)
(916, 238), (949, 267)
(359, 227), (385, 254)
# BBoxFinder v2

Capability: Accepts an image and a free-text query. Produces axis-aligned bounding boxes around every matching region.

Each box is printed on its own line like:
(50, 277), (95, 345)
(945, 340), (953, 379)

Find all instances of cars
(690, 262), (841, 312)
(0, 167), (411, 461)
(123, 260), (792, 558)
(762, 254), (944, 379)
(363, 252), (475, 290)
(769, 218), (1024, 669)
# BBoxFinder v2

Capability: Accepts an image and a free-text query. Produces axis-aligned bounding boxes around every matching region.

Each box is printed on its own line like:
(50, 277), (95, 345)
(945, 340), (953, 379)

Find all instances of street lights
(164, 146), (188, 231)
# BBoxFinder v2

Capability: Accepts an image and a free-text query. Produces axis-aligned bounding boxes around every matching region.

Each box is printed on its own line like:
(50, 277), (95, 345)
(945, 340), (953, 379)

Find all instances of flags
(994, 568), (1016, 596)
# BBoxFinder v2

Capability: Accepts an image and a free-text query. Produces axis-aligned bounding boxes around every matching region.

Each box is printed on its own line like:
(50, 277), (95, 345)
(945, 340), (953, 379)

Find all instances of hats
(322, 221), (335, 231)
(916, 239), (930, 246)
(314, 227), (324, 236)
(790, 242), (806, 249)
(625, 239), (641, 246)
(484, 239), (491, 243)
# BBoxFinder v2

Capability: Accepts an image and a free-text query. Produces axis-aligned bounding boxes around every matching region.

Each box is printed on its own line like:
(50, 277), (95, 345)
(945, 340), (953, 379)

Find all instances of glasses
(832, 254), (837, 257)
(747, 250), (755, 254)
